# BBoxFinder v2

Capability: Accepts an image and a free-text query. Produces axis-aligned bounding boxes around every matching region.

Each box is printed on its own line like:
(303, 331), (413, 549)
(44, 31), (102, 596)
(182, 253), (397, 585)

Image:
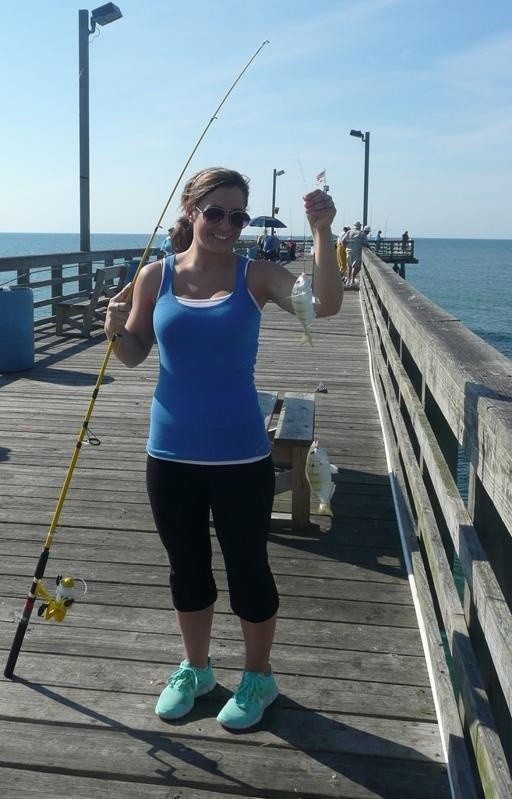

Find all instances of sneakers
(216, 670), (280, 730)
(341, 276), (358, 289)
(154, 655), (217, 721)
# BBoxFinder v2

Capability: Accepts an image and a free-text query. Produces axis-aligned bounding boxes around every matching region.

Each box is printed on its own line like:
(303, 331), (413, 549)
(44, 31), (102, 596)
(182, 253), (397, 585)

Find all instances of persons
(257, 229), (269, 260)
(287, 238), (296, 261)
(402, 230), (410, 254)
(375, 229), (384, 254)
(160, 226), (175, 258)
(264, 231), (279, 264)
(105, 164), (344, 731)
(337, 222), (371, 287)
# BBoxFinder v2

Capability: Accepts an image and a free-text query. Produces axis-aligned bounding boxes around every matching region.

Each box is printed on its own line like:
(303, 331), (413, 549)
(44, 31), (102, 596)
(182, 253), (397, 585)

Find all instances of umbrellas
(249, 216), (287, 230)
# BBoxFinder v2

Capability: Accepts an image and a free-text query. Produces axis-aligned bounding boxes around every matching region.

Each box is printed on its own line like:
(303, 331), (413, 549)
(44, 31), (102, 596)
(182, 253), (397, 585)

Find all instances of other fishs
(305, 440), (339, 518)
(287, 272), (322, 348)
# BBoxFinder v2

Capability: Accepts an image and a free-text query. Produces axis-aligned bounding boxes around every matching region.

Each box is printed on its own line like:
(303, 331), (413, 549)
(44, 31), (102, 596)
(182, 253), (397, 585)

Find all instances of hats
(343, 221), (371, 232)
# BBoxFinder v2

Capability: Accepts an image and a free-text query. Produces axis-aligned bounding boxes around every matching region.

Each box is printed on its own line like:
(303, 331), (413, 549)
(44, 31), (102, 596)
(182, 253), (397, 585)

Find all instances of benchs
(253, 390), (315, 532)
(54, 263), (128, 340)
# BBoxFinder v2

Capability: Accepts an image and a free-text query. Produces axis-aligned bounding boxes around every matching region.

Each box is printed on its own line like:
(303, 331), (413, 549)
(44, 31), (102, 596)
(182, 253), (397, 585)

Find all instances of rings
(327, 199), (333, 207)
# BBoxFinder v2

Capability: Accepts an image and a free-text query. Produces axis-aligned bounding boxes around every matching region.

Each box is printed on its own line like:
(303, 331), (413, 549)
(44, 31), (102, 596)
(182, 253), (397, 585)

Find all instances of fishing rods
(3, 40), (276, 677)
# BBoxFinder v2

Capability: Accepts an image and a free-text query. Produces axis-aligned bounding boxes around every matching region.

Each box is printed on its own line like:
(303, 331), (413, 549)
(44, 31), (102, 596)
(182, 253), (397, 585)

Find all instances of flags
(316, 169), (325, 182)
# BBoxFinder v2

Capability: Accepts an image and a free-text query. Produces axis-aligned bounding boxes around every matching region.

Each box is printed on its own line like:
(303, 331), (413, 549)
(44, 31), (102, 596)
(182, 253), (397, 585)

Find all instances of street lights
(350, 129), (370, 230)
(79, 1), (124, 291)
(271, 168), (285, 235)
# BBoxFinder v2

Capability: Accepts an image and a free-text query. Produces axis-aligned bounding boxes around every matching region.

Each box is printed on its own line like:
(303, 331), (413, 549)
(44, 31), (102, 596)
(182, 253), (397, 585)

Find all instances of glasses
(195, 207), (250, 229)
